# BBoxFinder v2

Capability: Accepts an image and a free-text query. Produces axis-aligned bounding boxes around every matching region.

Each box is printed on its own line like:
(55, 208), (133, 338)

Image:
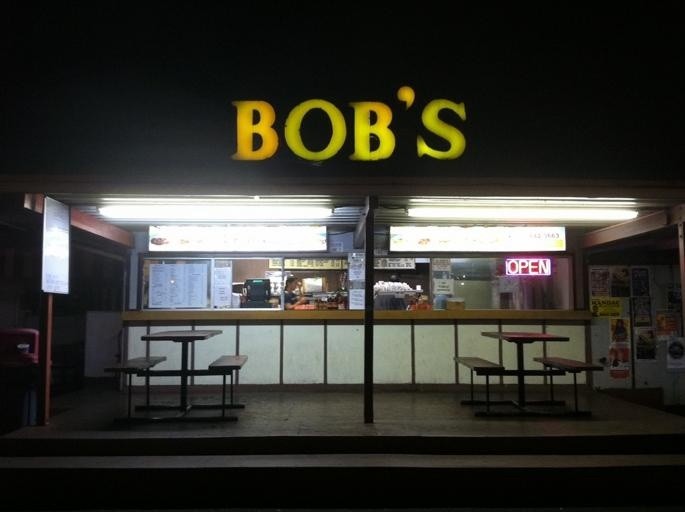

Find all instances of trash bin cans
(0, 328), (40, 427)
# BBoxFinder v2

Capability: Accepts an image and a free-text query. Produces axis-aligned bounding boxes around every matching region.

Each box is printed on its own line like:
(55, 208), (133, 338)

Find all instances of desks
(460, 332), (570, 420)
(112, 331), (245, 424)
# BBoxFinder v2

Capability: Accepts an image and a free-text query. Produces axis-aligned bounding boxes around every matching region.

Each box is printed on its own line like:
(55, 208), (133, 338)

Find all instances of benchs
(533, 357), (603, 374)
(208, 354), (248, 371)
(453, 355), (505, 373)
(103, 356), (166, 374)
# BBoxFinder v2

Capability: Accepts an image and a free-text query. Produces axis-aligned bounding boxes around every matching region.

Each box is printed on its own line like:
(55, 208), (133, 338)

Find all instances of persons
(278, 275), (307, 310)
(409, 290), (432, 310)
(373, 275), (406, 310)
(433, 293), (449, 309)
